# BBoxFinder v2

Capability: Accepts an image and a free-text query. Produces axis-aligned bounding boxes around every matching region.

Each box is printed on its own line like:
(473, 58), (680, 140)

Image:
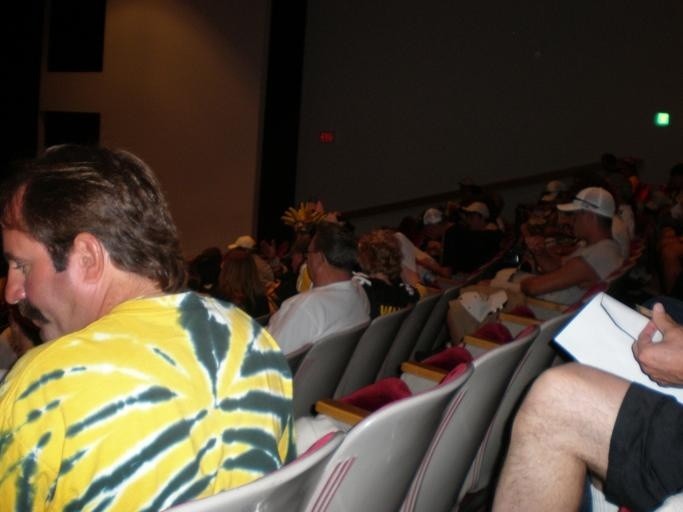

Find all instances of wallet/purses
(552, 290), (683, 405)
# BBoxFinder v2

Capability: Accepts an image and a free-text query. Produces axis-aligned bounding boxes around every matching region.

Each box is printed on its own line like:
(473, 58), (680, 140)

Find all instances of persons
(1, 142), (298, 512)
(190, 154), (682, 350)
(491, 302), (683, 512)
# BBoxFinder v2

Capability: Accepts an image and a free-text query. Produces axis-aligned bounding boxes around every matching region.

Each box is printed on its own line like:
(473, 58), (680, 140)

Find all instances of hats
(556, 187), (616, 218)
(227, 234), (257, 250)
(462, 202), (490, 219)
(541, 180), (567, 201)
(423, 208), (443, 225)
